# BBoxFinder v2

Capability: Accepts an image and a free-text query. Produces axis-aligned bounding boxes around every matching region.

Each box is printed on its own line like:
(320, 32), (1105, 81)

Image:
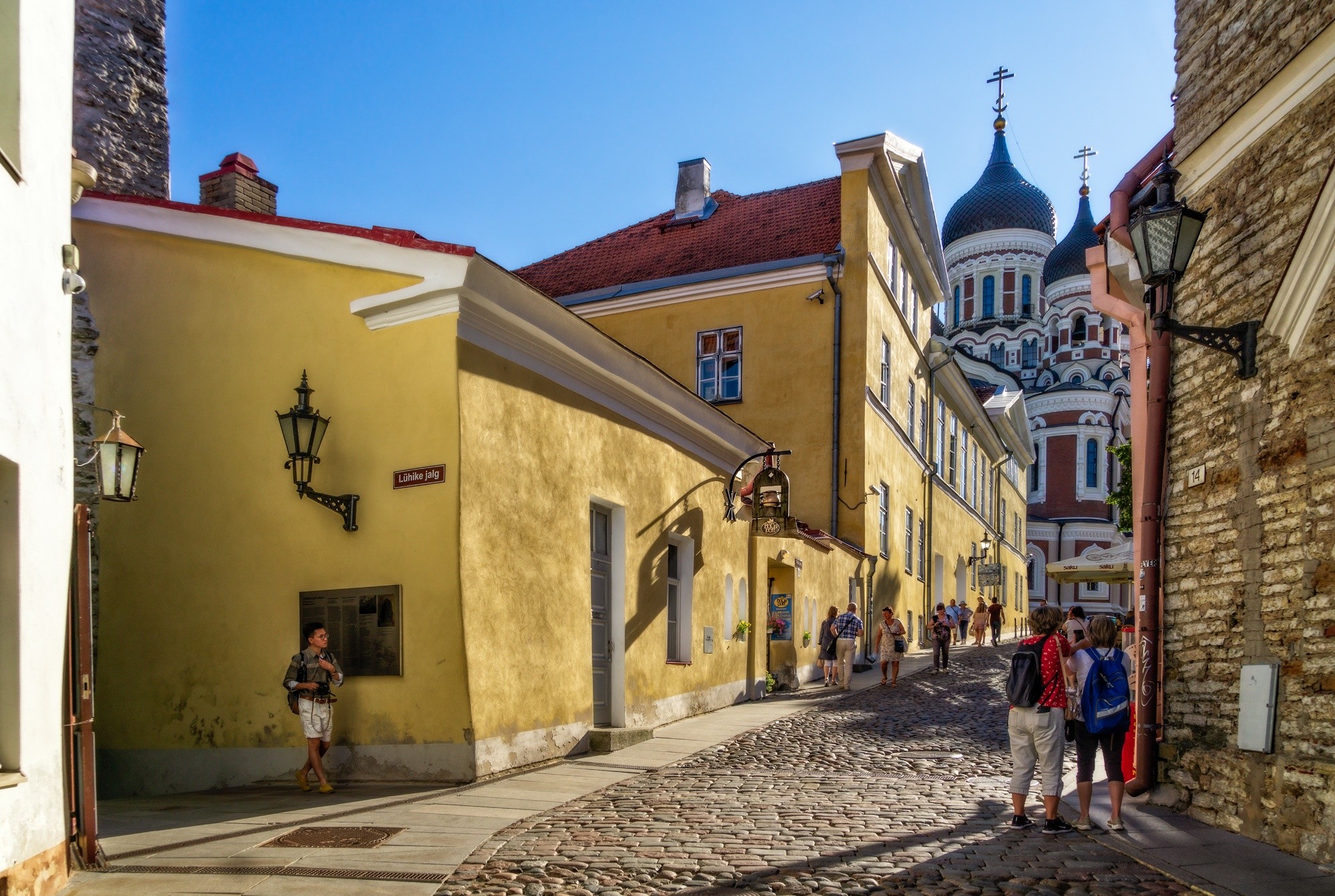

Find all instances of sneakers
(1107, 818), (1124, 830)
(1071, 817), (1091, 830)
(1011, 814), (1034, 829)
(1042, 817), (1073, 834)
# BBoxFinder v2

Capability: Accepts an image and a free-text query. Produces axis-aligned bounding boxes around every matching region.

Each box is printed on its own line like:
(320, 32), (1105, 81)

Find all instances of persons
(283, 622), (345, 794)
(1008, 599), (1135, 834)
(830, 602), (864, 691)
(943, 596), (1006, 648)
(927, 602), (956, 673)
(818, 605), (838, 687)
(875, 606), (906, 687)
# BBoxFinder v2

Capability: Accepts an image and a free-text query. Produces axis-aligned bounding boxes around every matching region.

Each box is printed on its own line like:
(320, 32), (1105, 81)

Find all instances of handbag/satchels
(866, 652), (878, 663)
(969, 625), (975, 636)
(1064, 696), (1078, 720)
(817, 660), (824, 668)
(902, 636), (908, 652)
(895, 640), (903, 652)
(1064, 720), (1074, 741)
(826, 639), (836, 654)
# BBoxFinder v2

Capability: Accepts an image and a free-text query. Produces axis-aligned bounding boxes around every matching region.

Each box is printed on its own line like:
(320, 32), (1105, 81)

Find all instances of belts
(300, 695), (337, 704)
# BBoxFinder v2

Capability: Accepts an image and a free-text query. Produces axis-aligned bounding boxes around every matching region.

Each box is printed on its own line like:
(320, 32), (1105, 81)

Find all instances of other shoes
(295, 769), (312, 792)
(839, 686), (850, 691)
(942, 668), (950, 673)
(319, 784), (334, 794)
(991, 639), (997, 646)
(931, 669), (939, 675)
(824, 680), (838, 687)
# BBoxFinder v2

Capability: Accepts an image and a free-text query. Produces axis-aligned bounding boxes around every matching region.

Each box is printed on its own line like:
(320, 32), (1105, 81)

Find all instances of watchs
(331, 670), (337, 676)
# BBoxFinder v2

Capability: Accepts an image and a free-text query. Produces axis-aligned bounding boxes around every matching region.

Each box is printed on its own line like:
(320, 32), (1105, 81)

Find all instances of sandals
(892, 682), (897, 688)
(881, 677), (887, 685)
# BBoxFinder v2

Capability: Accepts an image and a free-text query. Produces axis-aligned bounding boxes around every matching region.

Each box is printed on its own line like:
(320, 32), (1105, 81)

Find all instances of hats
(959, 600), (967, 607)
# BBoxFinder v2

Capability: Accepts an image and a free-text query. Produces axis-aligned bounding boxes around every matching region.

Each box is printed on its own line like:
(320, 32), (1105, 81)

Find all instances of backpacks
(1082, 647), (1131, 734)
(1006, 635), (1050, 707)
(288, 651), (332, 715)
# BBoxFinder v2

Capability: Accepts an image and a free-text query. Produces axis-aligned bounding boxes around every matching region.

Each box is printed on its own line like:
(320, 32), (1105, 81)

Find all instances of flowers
(765, 611), (785, 635)
(736, 619), (752, 634)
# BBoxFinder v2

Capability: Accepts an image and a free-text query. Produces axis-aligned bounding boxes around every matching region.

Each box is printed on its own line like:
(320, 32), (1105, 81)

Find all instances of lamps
(73, 400), (147, 502)
(274, 368), (361, 531)
(969, 529), (991, 566)
(724, 451), (794, 519)
(1124, 142), (1264, 380)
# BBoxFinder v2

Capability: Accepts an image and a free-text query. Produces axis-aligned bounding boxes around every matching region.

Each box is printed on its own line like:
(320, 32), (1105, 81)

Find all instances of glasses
(312, 634), (329, 639)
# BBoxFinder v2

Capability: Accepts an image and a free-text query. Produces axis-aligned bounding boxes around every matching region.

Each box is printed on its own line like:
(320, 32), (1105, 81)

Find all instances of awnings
(1044, 542), (1134, 584)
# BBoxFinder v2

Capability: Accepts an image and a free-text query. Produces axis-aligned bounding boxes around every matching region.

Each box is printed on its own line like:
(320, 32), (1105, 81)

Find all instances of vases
(766, 629), (773, 634)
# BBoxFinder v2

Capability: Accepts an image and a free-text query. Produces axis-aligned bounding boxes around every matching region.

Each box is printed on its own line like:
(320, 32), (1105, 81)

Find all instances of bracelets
(897, 632), (899, 636)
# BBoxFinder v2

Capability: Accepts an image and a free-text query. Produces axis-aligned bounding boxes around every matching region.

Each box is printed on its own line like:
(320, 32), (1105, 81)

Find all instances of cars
(1086, 615), (1117, 626)
